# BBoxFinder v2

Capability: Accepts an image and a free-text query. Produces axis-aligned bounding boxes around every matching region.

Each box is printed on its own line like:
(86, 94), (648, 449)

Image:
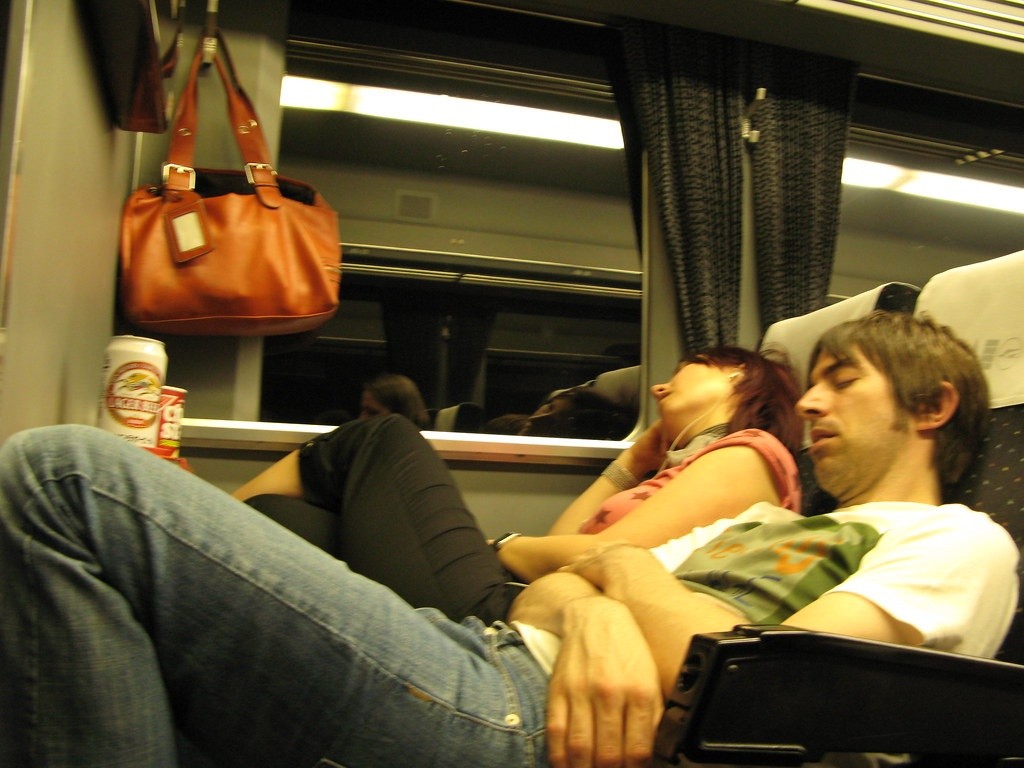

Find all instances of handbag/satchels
(118, 45), (342, 338)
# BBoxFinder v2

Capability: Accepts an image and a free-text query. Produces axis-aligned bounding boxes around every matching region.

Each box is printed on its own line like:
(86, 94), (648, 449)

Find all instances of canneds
(95, 335), (167, 448)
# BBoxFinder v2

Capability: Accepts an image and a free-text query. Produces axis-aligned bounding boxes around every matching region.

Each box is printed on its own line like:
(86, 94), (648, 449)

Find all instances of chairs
(433, 363), (642, 433)
(637, 251), (1024, 768)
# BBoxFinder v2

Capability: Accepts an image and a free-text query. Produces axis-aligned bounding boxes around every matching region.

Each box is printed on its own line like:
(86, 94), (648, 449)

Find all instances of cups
(157, 385), (189, 458)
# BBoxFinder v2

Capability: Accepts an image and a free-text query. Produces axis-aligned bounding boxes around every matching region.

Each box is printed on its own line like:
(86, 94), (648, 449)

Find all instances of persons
(360, 373), (431, 432)
(482, 390), (620, 444)
(0, 314), (1020, 768)
